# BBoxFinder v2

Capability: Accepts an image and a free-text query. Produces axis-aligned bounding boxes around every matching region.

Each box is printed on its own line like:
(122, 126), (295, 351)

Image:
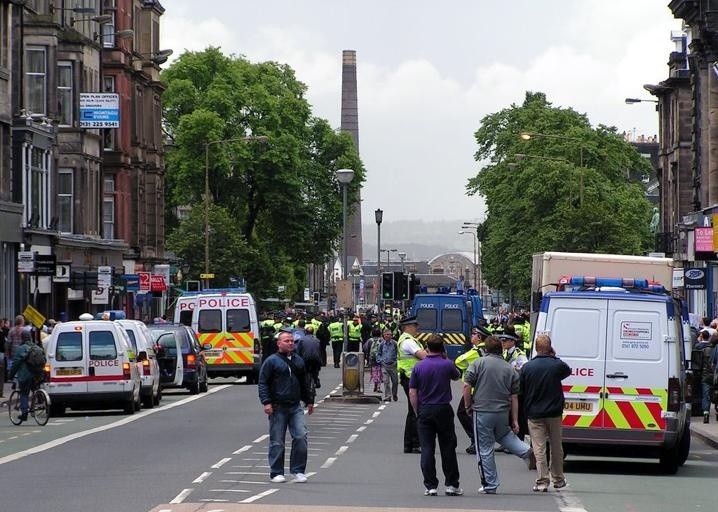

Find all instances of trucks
(527, 251), (674, 361)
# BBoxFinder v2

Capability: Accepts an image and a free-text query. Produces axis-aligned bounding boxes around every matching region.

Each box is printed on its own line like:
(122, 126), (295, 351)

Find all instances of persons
(454, 325), (492, 454)
(409, 335), (464, 496)
(258, 332), (315, 483)
(0, 315), (58, 421)
(520, 335), (572, 492)
(690, 317), (718, 423)
(479, 302), (531, 358)
(396, 316), (428, 454)
(494, 330), (529, 454)
(257, 307), (407, 402)
(464, 336), (536, 494)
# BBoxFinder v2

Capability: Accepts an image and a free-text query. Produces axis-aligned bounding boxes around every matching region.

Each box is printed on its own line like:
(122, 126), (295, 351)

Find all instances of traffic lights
(313, 291), (319, 307)
(401, 274), (408, 299)
(409, 276), (420, 300)
(381, 271), (395, 300)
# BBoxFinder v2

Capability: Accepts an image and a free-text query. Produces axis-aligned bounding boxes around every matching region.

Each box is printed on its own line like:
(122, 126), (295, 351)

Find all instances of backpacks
(27, 345), (46, 374)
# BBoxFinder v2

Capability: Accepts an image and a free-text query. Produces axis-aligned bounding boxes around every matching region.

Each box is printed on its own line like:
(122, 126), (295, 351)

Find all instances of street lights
(336, 168), (355, 393)
(507, 163), (519, 170)
(204, 135), (270, 288)
(623, 97), (659, 105)
(399, 252), (406, 317)
(449, 220), (484, 305)
(336, 233), (357, 280)
(379, 248), (397, 271)
(373, 209), (383, 337)
(521, 132), (583, 210)
(513, 153), (573, 210)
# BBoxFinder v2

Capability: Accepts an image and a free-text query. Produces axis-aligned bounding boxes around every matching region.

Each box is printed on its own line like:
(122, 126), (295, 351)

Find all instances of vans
(42, 313), (148, 416)
(190, 290), (266, 385)
(437, 287), (485, 348)
(409, 287), (474, 363)
(529, 277), (704, 475)
(173, 291), (198, 328)
(96, 310), (165, 408)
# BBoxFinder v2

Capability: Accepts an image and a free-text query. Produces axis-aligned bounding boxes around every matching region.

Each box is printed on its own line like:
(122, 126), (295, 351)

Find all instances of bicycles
(8, 374), (52, 426)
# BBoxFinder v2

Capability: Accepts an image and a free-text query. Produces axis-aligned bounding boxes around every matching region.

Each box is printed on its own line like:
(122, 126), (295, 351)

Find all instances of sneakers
(533, 484), (548, 491)
(290, 472), (307, 483)
(269, 474), (286, 483)
(424, 488), (437, 496)
(553, 479), (568, 488)
(445, 486), (464, 495)
(524, 449), (535, 470)
(479, 487), (496, 494)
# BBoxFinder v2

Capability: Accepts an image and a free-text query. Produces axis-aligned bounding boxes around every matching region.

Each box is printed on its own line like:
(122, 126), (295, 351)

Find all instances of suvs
(143, 322), (212, 396)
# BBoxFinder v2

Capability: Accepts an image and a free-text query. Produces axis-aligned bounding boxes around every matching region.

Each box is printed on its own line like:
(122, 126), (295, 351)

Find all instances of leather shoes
(703, 415), (709, 424)
(403, 446), (421, 454)
(466, 441), (477, 454)
(17, 414), (27, 421)
(494, 444), (510, 455)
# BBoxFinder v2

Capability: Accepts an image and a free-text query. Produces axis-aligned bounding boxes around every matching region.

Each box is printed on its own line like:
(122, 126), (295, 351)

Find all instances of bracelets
(464, 405), (471, 409)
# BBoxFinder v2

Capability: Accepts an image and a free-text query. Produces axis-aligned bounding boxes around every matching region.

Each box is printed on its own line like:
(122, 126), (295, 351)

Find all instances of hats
(499, 329), (519, 341)
(399, 316), (418, 325)
(470, 325), (491, 336)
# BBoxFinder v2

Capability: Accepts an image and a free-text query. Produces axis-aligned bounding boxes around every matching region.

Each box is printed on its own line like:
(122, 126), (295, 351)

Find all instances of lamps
(47, 216), (59, 230)
(625, 98), (659, 104)
(28, 214), (39, 228)
